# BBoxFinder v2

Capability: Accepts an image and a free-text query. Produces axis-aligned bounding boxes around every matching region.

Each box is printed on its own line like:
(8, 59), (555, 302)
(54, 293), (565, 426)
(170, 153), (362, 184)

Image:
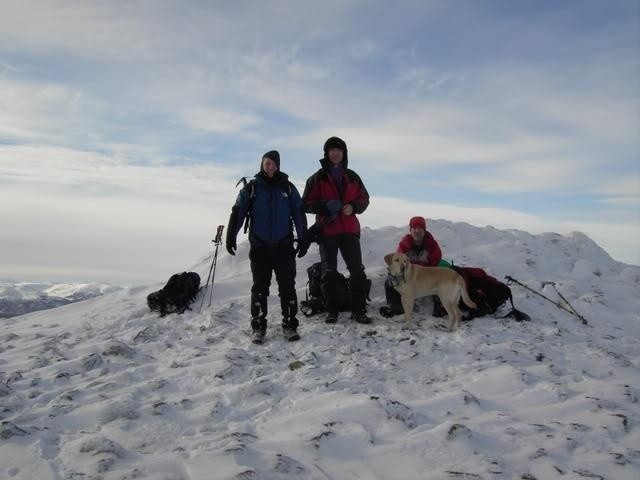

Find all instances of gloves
(324, 199), (342, 224)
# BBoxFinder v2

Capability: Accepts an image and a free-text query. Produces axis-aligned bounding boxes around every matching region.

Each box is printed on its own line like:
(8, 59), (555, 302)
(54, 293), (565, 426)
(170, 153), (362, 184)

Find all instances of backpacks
(300, 263), (352, 317)
(450, 266), (511, 318)
(147, 272), (200, 317)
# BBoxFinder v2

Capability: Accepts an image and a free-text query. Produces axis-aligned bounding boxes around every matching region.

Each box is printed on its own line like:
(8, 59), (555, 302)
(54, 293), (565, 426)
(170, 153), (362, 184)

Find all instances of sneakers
(251, 332), (264, 344)
(352, 312), (373, 323)
(295, 234), (312, 257)
(325, 311), (338, 324)
(226, 232), (236, 254)
(432, 304), (448, 317)
(379, 303), (403, 317)
(283, 329), (300, 340)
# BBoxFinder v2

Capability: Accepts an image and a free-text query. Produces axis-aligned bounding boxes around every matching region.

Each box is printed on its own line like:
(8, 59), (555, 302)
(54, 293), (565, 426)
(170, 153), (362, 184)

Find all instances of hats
(323, 137), (348, 169)
(409, 217), (428, 233)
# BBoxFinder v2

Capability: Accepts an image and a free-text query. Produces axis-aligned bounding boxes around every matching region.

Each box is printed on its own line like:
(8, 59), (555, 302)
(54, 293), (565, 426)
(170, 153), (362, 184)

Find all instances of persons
(380, 216), (448, 320)
(224, 150), (310, 346)
(303, 133), (376, 326)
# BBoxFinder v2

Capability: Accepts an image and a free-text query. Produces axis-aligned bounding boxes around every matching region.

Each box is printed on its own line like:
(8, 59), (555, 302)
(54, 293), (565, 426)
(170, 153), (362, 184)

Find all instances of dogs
(384, 252), (476, 333)
